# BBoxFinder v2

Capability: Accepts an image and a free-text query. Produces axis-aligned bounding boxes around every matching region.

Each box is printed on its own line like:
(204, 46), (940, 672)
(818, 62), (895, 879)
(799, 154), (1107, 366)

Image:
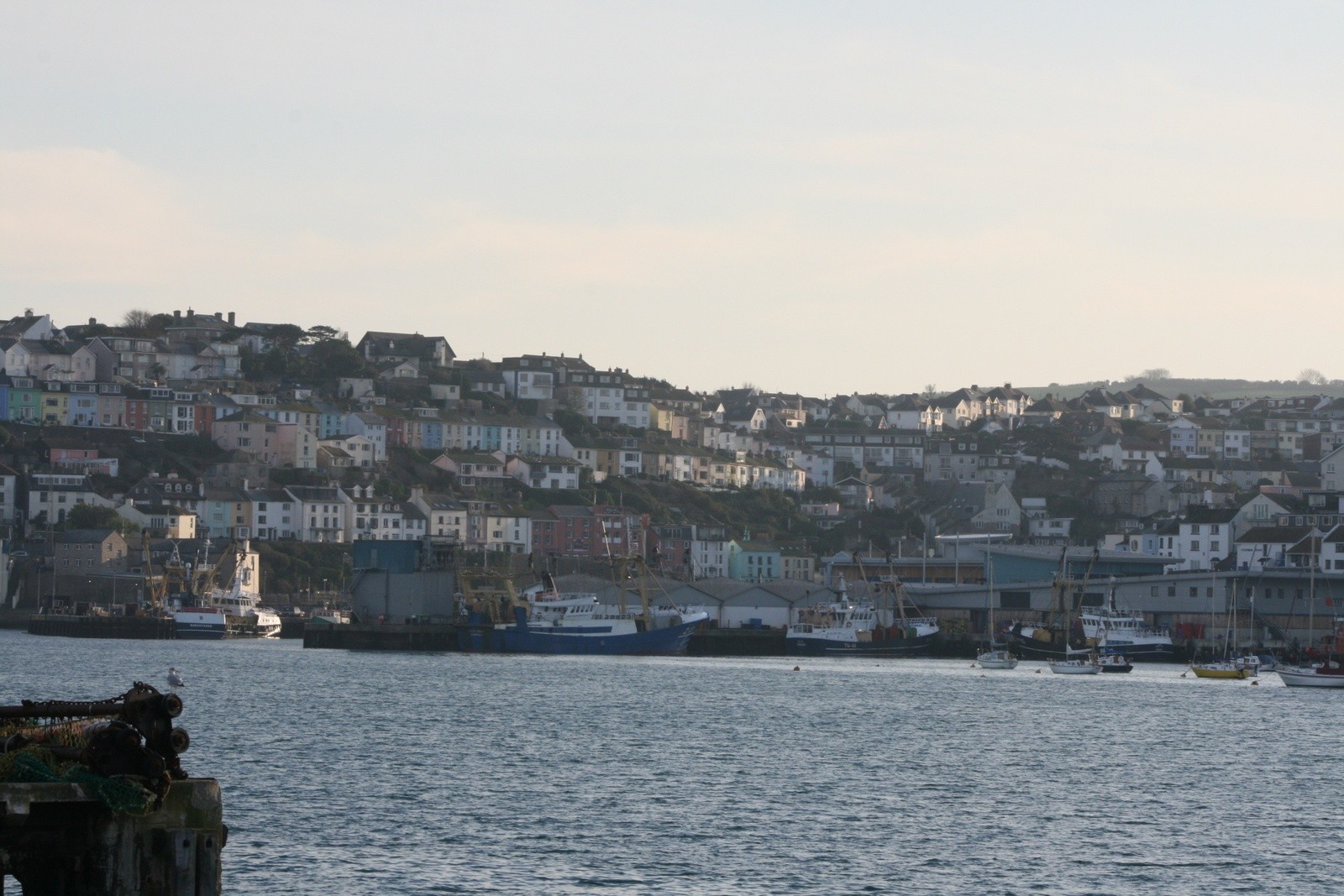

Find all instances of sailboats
(1210, 576), (1260, 677)
(1230, 585), (1282, 671)
(995, 536), (1174, 661)
(976, 556), (1019, 669)
(1047, 561), (1104, 675)
(1271, 523), (1344, 688)
(1189, 572), (1252, 679)
(1091, 584), (1134, 673)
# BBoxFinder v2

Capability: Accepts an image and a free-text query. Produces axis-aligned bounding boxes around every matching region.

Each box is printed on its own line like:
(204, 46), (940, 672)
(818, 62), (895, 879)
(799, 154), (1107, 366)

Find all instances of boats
(0, 516), (941, 657)
